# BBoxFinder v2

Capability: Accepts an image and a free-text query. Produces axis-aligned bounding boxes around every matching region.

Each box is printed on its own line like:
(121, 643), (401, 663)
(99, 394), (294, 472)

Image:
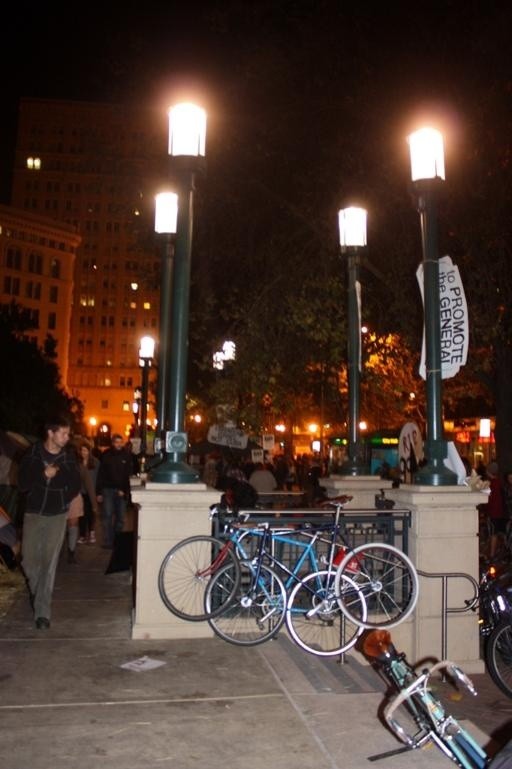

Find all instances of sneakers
(76, 531), (96, 544)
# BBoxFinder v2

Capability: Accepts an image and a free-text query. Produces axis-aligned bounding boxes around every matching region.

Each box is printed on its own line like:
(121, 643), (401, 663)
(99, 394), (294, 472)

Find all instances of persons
(481, 459), (502, 563)
(1, 431), (140, 570)
(16, 409), (82, 628)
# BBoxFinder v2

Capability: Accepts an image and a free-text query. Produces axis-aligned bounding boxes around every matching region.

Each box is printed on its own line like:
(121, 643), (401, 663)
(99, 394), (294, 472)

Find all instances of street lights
(338, 205), (369, 472)
(406, 126), (459, 486)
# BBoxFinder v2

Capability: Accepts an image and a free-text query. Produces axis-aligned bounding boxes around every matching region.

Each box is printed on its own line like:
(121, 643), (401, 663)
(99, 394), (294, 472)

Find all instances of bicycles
(363, 629), (492, 769)
(467, 568), (512, 699)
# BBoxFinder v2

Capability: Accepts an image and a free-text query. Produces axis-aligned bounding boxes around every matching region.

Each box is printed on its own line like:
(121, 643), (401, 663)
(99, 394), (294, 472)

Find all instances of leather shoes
(36, 617), (50, 630)
